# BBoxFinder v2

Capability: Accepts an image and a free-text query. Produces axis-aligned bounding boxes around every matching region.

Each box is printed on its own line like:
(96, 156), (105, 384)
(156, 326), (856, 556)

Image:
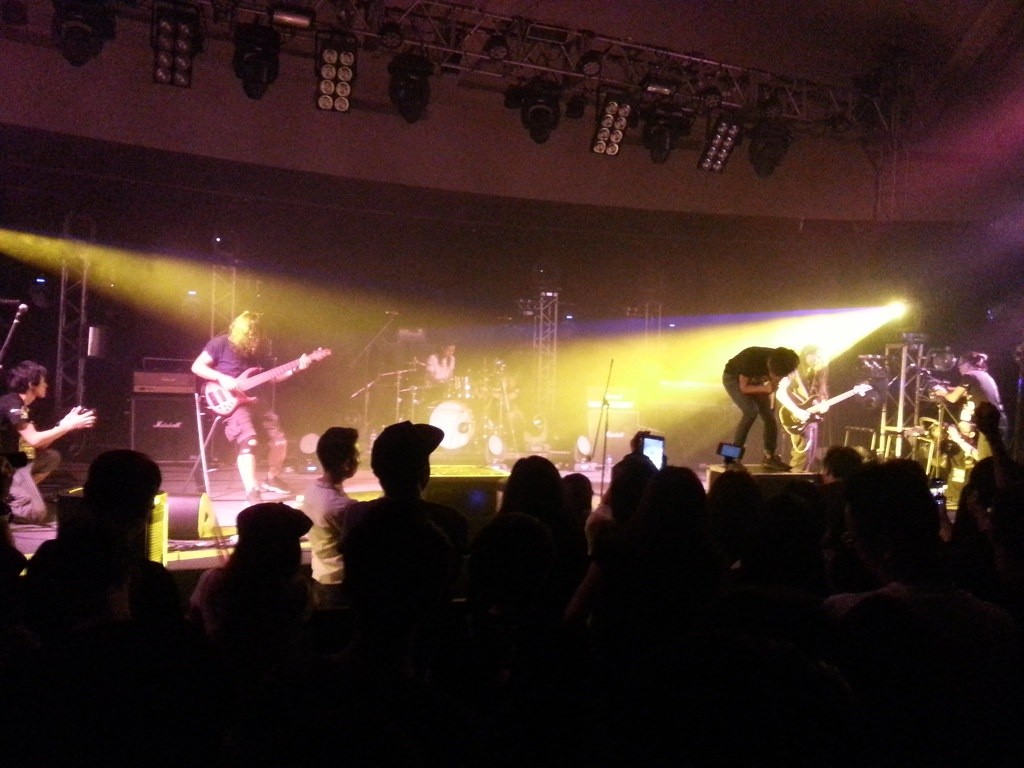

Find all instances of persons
(425, 339), (456, 387)
(25, 449), (206, 649)
(191, 310), (311, 507)
(946, 408), (992, 508)
(722, 346), (800, 472)
(0, 360), (97, 523)
(776, 344), (829, 472)
(301, 427), (362, 585)
(933, 352), (1009, 437)
(338, 401), (1024, 768)
(190, 502), (331, 648)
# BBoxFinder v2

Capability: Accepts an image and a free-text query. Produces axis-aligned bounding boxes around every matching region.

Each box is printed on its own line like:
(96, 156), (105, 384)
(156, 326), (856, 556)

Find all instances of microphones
(386, 311), (399, 315)
(770, 390), (776, 412)
(14, 303), (27, 324)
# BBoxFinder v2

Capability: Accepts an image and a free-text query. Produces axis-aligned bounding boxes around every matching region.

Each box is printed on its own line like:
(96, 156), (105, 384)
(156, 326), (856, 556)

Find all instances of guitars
(201, 347), (331, 416)
(780, 383), (873, 433)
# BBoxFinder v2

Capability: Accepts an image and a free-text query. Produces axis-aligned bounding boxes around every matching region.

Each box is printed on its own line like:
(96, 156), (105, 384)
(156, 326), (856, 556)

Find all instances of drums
(445, 376), (469, 399)
(428, 401), (474, 451)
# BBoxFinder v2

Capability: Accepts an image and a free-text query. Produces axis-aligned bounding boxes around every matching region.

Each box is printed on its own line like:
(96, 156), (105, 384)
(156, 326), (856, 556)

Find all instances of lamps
(591, 84), (792, 177)
(576, 53), (602, 77)
(382, 21), (404, 49)
(48, 0), (121, 69)
(483, 36), (511, 63)
(388, 53), (430, 122)
(312, 29), (359, 114)
(507, 76), (562, 145)
(230, 23), (282, 101)
(151, 0), (207, 86)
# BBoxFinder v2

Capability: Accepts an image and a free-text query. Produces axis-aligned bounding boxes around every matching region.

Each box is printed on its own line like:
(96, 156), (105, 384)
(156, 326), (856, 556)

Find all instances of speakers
(129, 395), (207, 466)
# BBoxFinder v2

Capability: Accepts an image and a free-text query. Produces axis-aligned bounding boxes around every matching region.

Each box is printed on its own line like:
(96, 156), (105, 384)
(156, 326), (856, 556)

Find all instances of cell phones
(642, 435), (666, 470)
(716, 443), (746, 461)
(929, 477), (945, 506)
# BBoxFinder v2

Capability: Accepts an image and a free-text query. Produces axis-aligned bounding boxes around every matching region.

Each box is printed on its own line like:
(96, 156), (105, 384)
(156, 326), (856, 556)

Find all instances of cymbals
(405, 361), (428, 367)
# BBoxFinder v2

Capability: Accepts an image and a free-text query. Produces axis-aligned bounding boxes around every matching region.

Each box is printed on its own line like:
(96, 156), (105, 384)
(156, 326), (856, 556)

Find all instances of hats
(371, 421), (445, 473)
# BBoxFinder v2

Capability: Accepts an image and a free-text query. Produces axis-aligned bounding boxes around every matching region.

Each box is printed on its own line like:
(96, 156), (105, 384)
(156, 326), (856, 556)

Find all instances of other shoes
(263, 476), (292, 494)
(761, 453), (793, 472)
(724, 462), (747, 471)
(248, 488), (263, 505)
